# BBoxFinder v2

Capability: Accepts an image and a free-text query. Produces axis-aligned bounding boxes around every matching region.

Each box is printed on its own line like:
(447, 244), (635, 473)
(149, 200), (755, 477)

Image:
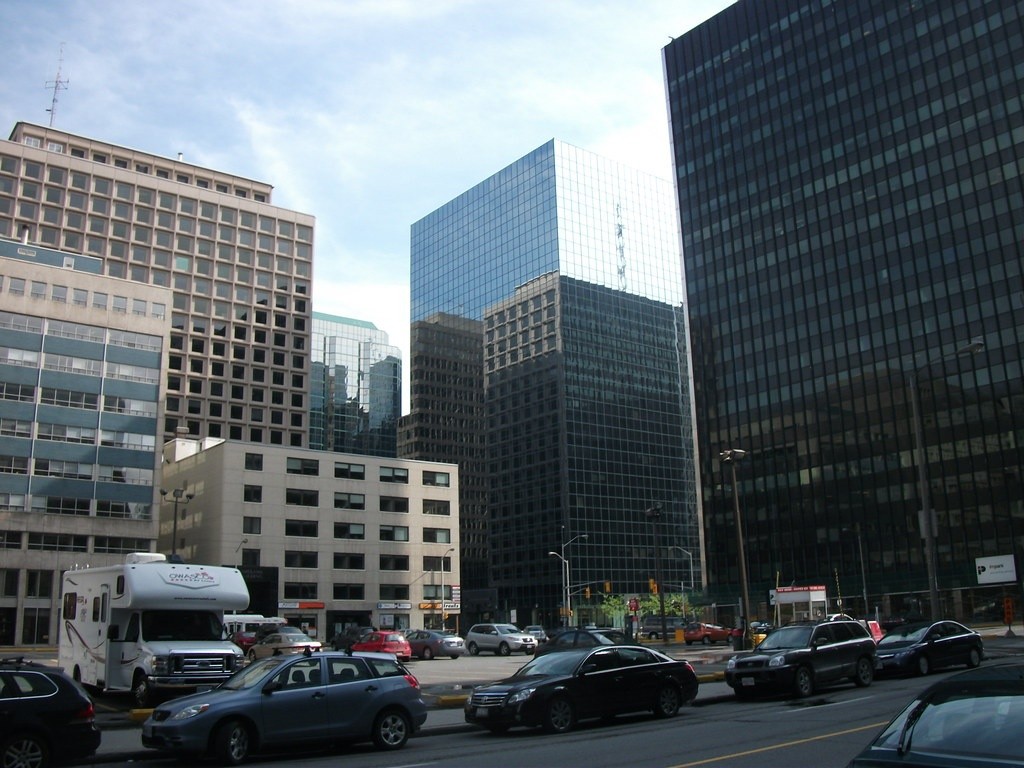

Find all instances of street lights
(233, 539), (248, 615)
(718, 448), (749, 620)
(441, 548), (455, 631)
(842, 523), (869, 617)
(561, 525), (588, 627)
(160, 488), (194, 554)
(548, 551), (571, 627)
(669, 546), (695, 618)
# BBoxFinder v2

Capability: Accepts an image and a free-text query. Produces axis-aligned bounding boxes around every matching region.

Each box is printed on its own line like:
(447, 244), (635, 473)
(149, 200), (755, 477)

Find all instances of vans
(639, 611), (778, 646)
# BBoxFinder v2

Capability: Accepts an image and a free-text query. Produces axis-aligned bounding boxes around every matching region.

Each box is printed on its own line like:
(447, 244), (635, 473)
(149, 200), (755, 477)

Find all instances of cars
(463, 646), (699, 735)
(872, 620), (984, 682)
(351, 632), (411, 662)
(845, 662), (1023, 768)
(222, 615), (323, 661)
(406, 630), (465, 660)
(535, 630), (665, 656)
(141, 648), (427, 767)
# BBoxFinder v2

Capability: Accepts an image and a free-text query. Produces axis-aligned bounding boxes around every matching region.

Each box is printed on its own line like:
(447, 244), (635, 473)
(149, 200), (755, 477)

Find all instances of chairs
(309, 669), (320, 686)
(340, 668), (354, 682)
(292, 671), (305, 686)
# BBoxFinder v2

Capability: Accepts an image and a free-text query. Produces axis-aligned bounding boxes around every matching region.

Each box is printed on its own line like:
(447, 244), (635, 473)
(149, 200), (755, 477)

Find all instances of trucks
(57, 553), (249, 709)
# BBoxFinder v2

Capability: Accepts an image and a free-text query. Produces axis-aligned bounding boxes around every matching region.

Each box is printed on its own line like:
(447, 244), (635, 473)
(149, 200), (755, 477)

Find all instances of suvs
(0, 656), (101, 768)
(330, 627), (377, 651)
(465, 624), (546, 657)
(724, 620), (878, 699)
